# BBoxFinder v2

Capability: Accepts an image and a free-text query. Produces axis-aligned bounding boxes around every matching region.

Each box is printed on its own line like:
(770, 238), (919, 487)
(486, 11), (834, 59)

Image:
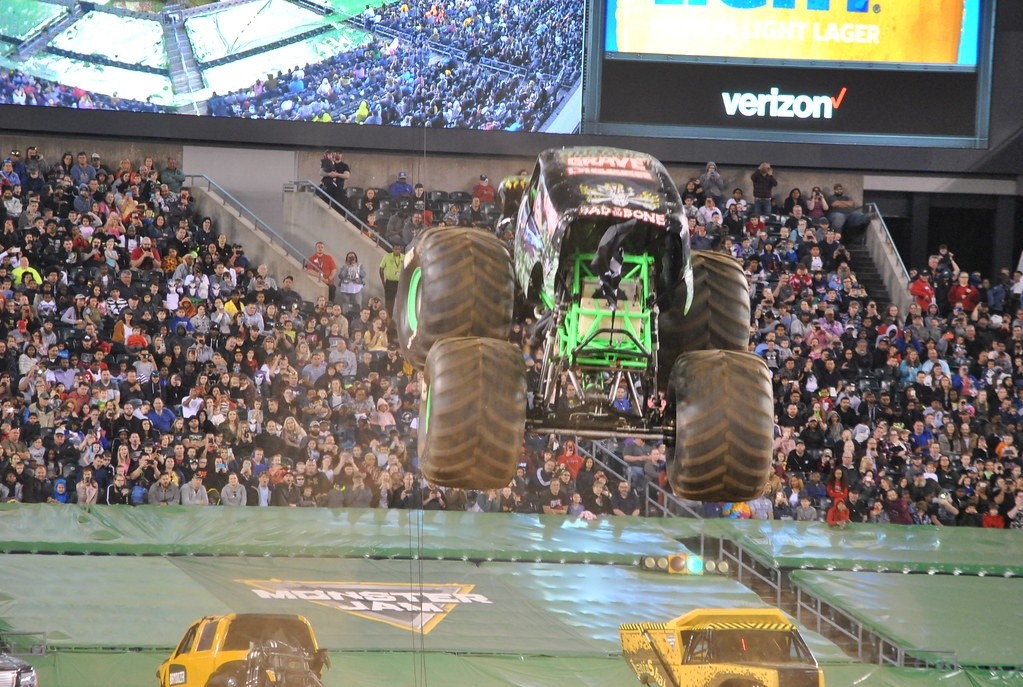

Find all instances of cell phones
(146, 459), (154, 465)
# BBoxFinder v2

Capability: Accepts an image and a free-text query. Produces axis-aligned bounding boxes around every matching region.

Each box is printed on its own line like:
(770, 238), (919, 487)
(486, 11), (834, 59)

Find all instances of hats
(91, 153), (100, 158)
(817, 296), (854, 328)
(440, 70), (451, 79)
(249, 107), (254, 113)
(40, 392), (65, 434)
(824, 449), (832, 458)
(74, 294), (141, 341)
(808, 417), (816, 422)
(340, 114), (346, 121)
(819, 217), (828, 224)
(977, 457), (985, 463)
(151, 371), (160, 377)
(267, 74), (273, 78)
(911, 469), (926, 475)
(919, 268), (930, 276)
(333, 147), (488, 208)
(978, 312), (990, 321)
(80, 187), (88, 191)
(210, 323), (260, 330)
(190, 459), (202, 478)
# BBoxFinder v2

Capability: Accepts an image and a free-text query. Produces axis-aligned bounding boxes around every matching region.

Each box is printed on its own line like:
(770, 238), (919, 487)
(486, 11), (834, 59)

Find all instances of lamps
(639, 553), (729, 575)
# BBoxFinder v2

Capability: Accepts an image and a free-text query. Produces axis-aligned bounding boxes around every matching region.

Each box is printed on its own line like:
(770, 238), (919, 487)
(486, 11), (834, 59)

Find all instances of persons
(0, 147), (1023, 529)
(0, 66), (166, 114)
(324, 1), (332, 7)
(208, 0), (585, 132)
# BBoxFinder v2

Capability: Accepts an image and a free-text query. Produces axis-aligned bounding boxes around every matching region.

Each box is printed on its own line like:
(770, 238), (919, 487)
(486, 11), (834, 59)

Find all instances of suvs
(0, 640), (36, 687)
(159, 615), (329, 687)
(619, 609), (822, 687)
(395, 147), (772, 501)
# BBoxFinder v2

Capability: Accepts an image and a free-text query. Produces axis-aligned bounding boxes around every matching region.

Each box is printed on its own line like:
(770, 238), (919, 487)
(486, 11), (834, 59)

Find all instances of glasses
(10, 150), (20, 156)
(266, 340), (274, 343)
(92, 158), (99, 161)
(3, 160), (12, 162)
(843, 281), (851, 283)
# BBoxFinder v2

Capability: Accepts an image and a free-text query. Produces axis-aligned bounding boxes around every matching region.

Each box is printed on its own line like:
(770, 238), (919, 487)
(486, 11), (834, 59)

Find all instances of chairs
(842, 368), (906, 415)
(345, 187), (500, 240)
(206, 41), (429, 128)
(762, 212), (784, 248)
